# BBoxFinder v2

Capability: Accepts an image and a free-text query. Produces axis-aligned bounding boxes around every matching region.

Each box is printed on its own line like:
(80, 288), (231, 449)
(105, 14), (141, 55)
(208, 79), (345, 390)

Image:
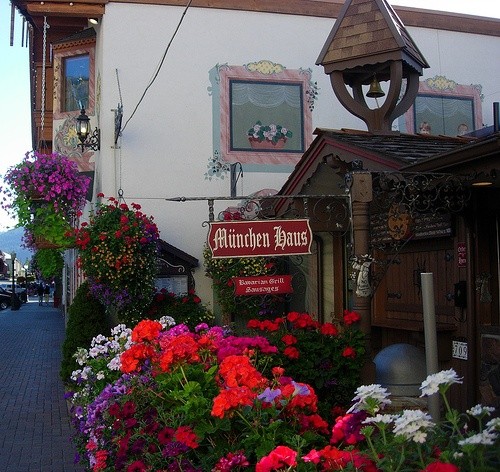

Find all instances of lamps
(74, 105), (100, 154)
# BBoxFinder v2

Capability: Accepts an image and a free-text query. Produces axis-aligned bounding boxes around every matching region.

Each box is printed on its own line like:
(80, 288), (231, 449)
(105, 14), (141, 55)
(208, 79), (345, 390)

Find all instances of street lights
(10, 250), (17, 309)
(23, 264), (29, 289)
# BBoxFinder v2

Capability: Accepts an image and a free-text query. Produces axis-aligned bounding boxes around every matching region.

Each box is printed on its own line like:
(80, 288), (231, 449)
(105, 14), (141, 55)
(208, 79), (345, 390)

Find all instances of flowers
(0, 149), (161, 323)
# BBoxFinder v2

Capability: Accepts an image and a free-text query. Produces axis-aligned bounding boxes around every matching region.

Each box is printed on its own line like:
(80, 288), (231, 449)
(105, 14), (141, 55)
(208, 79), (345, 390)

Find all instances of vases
(35, 235), (74, 249)
(31, 190), (44, 200)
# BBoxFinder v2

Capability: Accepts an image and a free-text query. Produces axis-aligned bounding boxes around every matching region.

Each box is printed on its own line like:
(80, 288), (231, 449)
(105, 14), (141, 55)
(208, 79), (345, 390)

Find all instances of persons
(35, 283), (51, 307)
(419, 122), (432, 134)
(457, 124), (469, 135)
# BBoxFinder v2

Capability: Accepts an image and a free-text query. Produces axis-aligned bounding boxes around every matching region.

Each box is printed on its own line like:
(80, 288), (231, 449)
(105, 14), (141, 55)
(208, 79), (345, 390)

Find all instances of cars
(0, 283), (27, 310)
